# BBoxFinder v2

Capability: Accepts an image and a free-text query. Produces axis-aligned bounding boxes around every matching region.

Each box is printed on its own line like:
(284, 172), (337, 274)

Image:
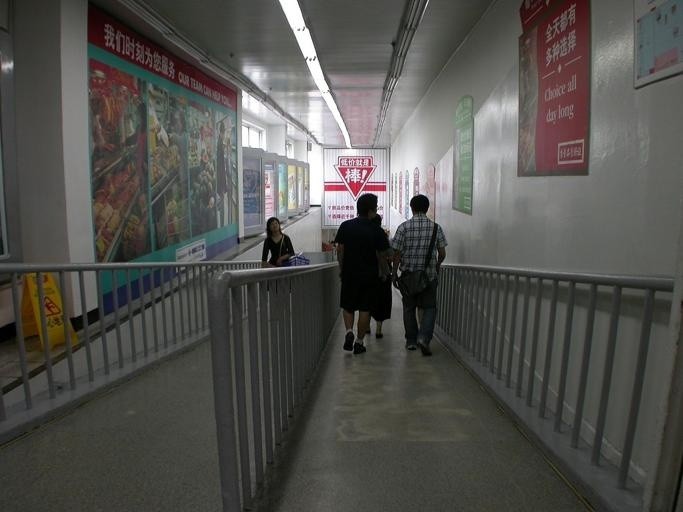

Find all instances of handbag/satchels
(397, 269), (430, 302)
(376, 254), (392, 281)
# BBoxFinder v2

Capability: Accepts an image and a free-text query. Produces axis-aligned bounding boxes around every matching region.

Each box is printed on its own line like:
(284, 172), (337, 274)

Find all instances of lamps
(278, 0), (352, 149)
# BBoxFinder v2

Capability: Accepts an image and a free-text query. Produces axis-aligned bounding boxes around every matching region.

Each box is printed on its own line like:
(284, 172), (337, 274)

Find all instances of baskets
(285, 256), (311, 266)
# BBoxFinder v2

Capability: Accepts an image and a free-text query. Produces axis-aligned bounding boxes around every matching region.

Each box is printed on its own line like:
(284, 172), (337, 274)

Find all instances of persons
(389, 193), (448, 357)
(87, 79), (232, 227)
(365, 214), (389, 338)
(261, 217), (297, 324)
(332, 193), (392, 355)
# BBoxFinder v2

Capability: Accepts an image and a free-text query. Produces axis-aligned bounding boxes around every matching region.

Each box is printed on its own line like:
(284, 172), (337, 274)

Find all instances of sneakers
(366, 329), (371, 334)
(405, 343), (417, 351)
(416, 337), (433, 356)
(353, 341), (366, 354)
(342, 332), (355, 351)
(376, 332), (383, 339)
